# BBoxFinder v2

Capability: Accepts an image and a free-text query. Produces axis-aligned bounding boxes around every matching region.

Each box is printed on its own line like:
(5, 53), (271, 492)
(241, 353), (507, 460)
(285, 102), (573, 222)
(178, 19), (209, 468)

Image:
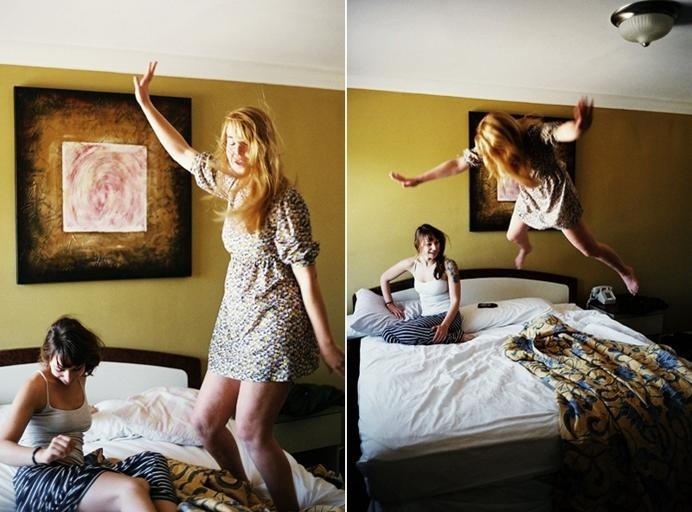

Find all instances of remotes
(478, 303), (497, 307)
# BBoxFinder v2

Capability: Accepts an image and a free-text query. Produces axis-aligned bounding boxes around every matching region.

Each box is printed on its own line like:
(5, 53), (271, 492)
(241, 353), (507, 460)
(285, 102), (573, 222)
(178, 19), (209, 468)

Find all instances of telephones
(592, 286), (616, 305)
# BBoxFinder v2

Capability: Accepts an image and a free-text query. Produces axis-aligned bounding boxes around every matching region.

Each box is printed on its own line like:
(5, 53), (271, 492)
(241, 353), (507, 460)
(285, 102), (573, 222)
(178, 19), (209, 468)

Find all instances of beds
(0, 344), (344, 512)
(355, 266), (692, 500)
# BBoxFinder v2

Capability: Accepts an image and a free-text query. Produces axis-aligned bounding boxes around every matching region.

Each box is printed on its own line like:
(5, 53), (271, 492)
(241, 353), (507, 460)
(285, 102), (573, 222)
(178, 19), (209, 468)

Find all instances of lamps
(611, 2), (682, 47)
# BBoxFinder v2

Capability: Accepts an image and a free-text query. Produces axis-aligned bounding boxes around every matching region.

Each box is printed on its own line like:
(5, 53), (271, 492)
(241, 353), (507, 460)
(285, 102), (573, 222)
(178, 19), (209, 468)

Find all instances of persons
(389, 97), (638, 298)
(131, 57), (343, 511)
(381, 226), (477, 345)
(1, 318), (181, 511)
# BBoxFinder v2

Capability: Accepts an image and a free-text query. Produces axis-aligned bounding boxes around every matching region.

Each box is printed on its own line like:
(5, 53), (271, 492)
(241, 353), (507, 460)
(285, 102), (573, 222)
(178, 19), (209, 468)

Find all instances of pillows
(351, 290), (420, 335)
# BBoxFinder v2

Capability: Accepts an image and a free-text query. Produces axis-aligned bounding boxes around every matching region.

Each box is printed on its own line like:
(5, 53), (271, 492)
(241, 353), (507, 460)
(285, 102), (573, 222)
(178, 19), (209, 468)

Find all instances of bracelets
(385, 301), (393, 306)
(31, 446), (42, 466)
